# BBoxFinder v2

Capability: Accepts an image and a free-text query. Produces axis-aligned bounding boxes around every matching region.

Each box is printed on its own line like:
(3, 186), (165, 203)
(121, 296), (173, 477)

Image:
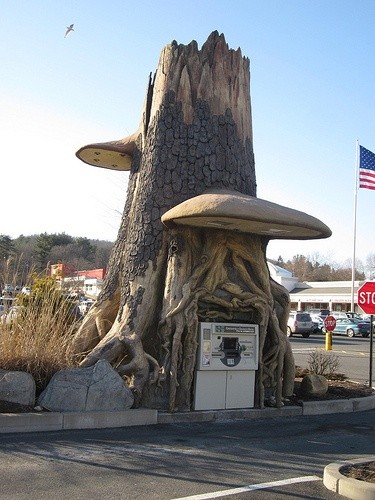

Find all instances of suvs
(287, 310), (314, 338)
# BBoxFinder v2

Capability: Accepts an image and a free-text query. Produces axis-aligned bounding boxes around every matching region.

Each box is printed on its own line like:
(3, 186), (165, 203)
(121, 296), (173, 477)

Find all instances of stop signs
(323, 316), (336, 331)
(357, 281), (375, 314)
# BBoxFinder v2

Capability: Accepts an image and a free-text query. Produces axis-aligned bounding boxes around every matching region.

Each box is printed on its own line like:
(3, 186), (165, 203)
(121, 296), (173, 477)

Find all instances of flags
(358, 144), (375, 191)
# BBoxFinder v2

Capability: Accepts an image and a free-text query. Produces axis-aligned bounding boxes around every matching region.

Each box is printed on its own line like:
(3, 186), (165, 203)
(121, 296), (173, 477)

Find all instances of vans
(327, 311), (363, 322)
(303, 308), (330, 323)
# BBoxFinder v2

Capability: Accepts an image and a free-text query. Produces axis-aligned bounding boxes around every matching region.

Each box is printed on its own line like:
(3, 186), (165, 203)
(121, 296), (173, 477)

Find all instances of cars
(1, 282), (96, 325)
(359, 314), (375, 334)
(320, 318), (371, 337)
(311, 314), (327, 333)
